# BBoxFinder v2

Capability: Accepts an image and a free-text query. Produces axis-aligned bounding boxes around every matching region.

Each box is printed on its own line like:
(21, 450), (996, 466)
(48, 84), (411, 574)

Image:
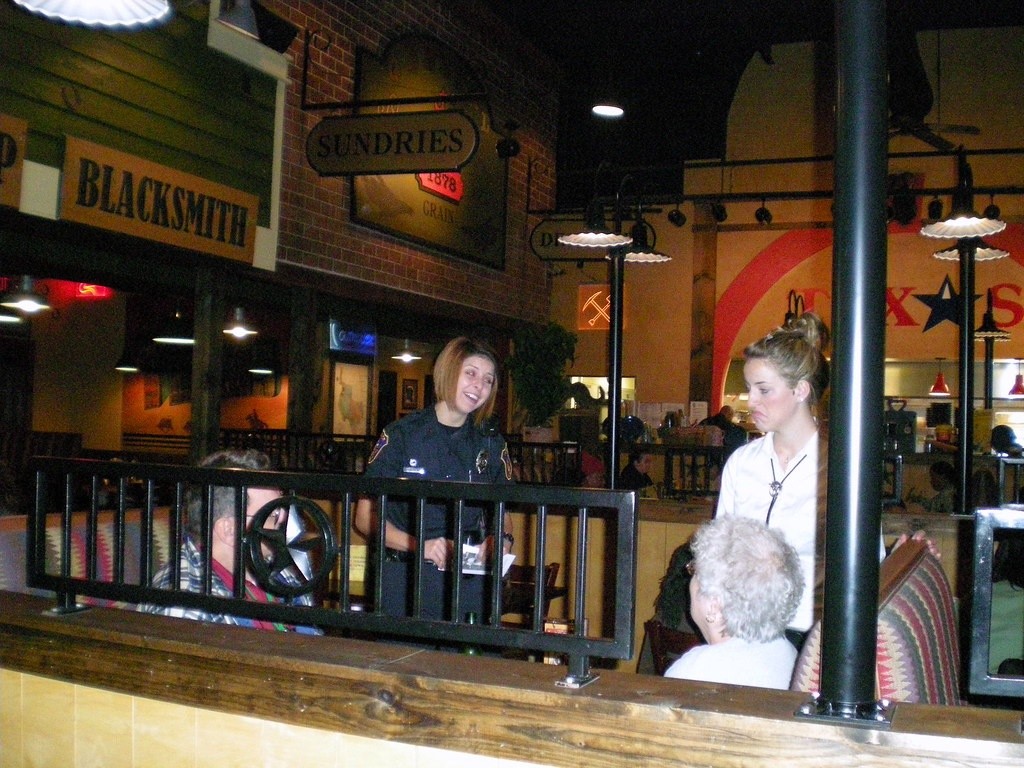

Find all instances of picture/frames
(402, 379), (418, 409)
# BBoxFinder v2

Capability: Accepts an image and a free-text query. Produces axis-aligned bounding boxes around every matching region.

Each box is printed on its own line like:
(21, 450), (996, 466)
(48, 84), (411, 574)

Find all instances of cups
(937, 424), (951, 442)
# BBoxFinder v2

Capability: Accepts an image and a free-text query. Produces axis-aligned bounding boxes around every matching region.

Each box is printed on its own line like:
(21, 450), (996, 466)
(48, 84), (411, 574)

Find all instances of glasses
(244, 508), (282, 525)
(685, 557), (697, 577)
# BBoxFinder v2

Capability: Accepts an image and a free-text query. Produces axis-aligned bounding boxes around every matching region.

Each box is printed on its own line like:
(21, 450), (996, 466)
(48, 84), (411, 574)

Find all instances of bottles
(462, 612), (481, 656)
(664, 411), (676, 428)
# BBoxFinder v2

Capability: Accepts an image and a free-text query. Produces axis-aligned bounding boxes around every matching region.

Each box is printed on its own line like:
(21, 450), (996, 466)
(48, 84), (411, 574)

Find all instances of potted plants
(504, 321), (577, 484)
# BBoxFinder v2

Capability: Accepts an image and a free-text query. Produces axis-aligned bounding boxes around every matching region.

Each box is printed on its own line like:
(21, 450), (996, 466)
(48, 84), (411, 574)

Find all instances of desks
(620, 442), (725, 498)
(899, 451), (1024, 507)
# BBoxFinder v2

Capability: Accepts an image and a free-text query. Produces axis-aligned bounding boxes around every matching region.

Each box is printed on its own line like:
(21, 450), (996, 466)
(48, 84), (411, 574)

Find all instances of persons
(136, 448), (328, 636)
(953, 529), (1024, 677)
(355, 336), (516, 650)
(693, 406), (735, 458)
(913, 462), (1002, 515)
(664, 517), (806, 691)
(716, 315), (942, 652)
(616, 451), (655, 490)
(638, 543), (706, 676)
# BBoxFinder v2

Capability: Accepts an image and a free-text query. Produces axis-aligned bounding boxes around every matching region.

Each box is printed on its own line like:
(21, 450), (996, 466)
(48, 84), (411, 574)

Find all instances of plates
(926, 402), (952, 428)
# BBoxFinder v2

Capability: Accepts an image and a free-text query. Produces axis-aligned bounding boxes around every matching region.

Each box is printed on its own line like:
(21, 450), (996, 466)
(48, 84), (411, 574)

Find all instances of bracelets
(489, 530), (515, 545)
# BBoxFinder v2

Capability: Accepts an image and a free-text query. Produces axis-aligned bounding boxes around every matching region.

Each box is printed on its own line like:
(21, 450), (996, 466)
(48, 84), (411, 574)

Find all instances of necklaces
(766, 455), (806, 526)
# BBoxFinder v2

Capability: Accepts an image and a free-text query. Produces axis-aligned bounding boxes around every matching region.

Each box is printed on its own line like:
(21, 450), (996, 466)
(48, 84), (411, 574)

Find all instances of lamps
(221, 304), (258, 339)
(391, 339), (422, 362)
(153, 333), (195, 345)
(495, 119), (520, 158)
(755, 199), (773, 226)
(708, 201), (727, 222)
(928, 196), (942, 220)
(248, 362), (274, 375)
(115, 355), (138, 372)
(1009, 363), (1024, 395)
(982, 194), (1000, 220)
(667, 202), (687, 228)
(928, 357), (950, 396)
(0, 273), (50, 322)
